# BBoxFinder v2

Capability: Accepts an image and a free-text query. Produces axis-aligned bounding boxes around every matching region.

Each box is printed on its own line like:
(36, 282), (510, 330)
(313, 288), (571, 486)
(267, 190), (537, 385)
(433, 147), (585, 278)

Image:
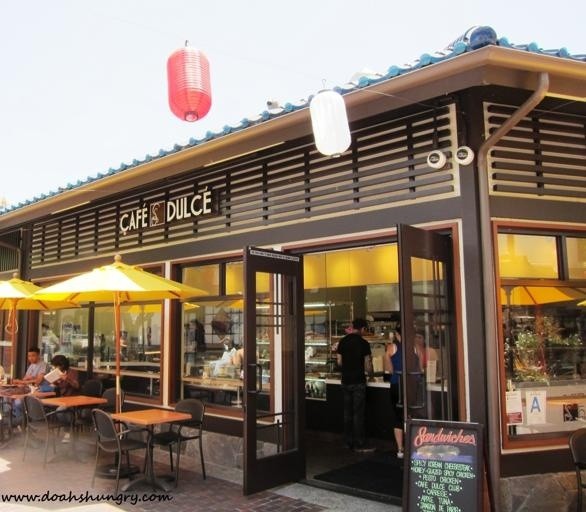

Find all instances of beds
(69, 352), (270, 408)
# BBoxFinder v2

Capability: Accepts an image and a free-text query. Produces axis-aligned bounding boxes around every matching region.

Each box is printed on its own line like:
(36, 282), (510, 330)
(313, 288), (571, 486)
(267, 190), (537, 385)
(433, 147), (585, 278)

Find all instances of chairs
(0, 373), (206, 501)
(569, 429), (586, 512)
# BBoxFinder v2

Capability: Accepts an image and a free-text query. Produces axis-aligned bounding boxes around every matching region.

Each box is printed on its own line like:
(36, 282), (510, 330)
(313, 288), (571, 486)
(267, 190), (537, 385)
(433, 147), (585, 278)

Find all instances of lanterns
(309, 86), (353, 163)
(167, 39), (214, 127)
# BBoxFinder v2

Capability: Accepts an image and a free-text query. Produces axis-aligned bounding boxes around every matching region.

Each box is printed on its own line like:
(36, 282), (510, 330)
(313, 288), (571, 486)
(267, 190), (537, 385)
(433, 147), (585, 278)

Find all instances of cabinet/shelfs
(254, 302), (354, 378)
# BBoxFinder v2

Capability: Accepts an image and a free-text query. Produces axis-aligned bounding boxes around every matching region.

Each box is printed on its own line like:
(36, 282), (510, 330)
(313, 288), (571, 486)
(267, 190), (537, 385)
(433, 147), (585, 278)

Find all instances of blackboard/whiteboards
(402, 419), (484, 512)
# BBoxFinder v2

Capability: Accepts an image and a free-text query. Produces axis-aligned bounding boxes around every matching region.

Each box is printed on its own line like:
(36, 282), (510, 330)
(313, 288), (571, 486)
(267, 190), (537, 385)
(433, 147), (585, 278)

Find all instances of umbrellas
(0, 272), (81, 384)
(498, 278), (586, 384)
(197, 294), (279, 388)
(31, 252), (211, 434)
(95, 300), (202, 364)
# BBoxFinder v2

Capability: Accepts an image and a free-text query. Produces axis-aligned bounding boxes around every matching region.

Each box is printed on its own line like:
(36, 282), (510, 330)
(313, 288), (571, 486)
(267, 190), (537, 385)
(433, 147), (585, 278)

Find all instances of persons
(386, 320), (425, 460)
(335, 317), (379, 454)
(219, 337), (237, 364)
(41, 322), (58, 350)
(41, 356), (79, 444)
(414, 327), (440, 383)
(12, 346), (49, 426)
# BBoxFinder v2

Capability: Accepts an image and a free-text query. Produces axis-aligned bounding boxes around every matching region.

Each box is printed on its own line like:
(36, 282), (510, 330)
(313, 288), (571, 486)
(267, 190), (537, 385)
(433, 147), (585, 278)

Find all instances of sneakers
(60, 431), (73, 443)
(346, 438), (405, 459)
